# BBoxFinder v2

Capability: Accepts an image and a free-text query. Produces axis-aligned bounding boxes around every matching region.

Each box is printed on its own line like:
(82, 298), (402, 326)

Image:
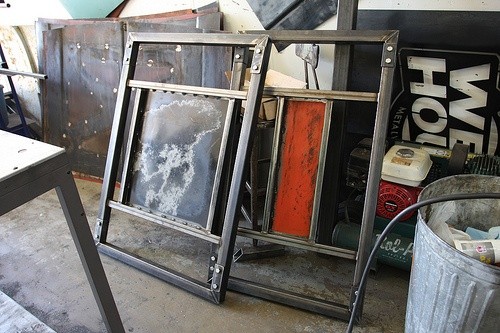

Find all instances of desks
(0, 128), (126, 333)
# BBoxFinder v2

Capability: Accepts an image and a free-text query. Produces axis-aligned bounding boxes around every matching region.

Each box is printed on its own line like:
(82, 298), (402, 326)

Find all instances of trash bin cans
(405, 174), (500, 333)
